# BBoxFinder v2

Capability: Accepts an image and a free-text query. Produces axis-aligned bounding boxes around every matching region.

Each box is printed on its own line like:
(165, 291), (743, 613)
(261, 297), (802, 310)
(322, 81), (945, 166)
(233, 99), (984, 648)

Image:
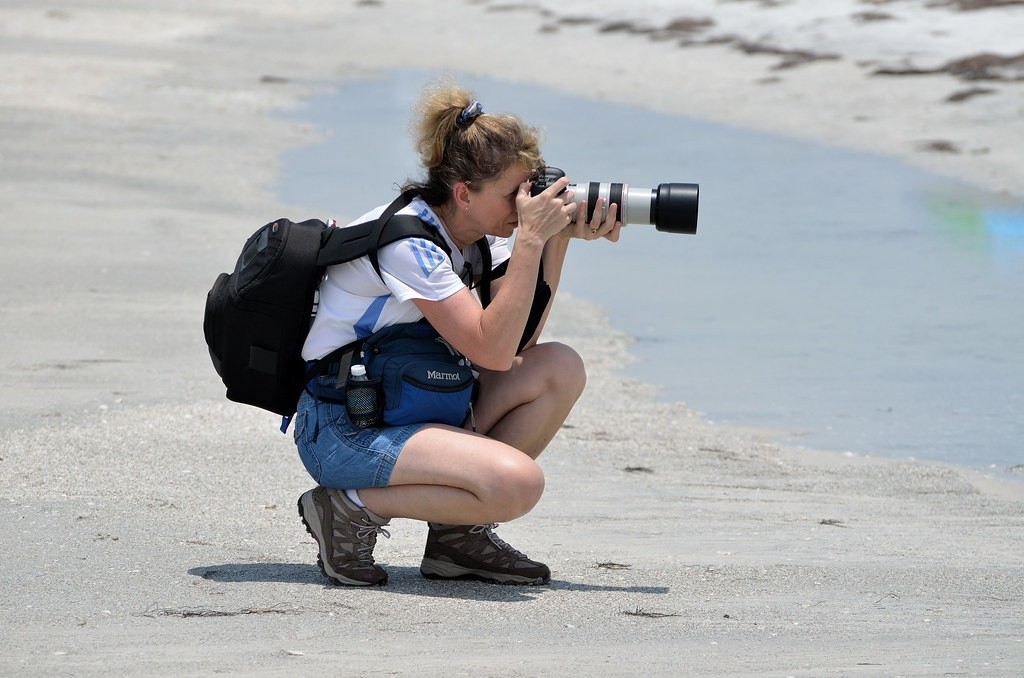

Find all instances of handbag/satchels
(344, 322), (479, 432)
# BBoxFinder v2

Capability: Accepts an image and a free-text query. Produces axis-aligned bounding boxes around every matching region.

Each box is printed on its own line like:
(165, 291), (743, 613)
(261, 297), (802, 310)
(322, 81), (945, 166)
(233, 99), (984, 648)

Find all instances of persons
(293, 91), (621, 587)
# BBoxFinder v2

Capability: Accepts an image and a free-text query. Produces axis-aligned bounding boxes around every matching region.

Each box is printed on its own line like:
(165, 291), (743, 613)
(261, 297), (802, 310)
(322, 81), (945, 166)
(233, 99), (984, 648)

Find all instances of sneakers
(297, 487), (391, 586)
(419, 522), (551, 585)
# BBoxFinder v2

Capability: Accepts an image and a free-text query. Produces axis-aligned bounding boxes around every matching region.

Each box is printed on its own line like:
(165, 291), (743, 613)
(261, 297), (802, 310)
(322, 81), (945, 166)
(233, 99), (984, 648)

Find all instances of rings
(591, 228), (598, 233)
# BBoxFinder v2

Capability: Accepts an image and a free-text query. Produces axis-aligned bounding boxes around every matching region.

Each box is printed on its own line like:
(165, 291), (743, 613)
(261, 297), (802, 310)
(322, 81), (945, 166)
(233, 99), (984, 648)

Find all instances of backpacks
(203, 218), (455, 417)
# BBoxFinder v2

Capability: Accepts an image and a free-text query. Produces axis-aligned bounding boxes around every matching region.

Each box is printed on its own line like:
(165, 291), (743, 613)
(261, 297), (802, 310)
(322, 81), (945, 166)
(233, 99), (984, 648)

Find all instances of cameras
(529, 166), (700, 235)
